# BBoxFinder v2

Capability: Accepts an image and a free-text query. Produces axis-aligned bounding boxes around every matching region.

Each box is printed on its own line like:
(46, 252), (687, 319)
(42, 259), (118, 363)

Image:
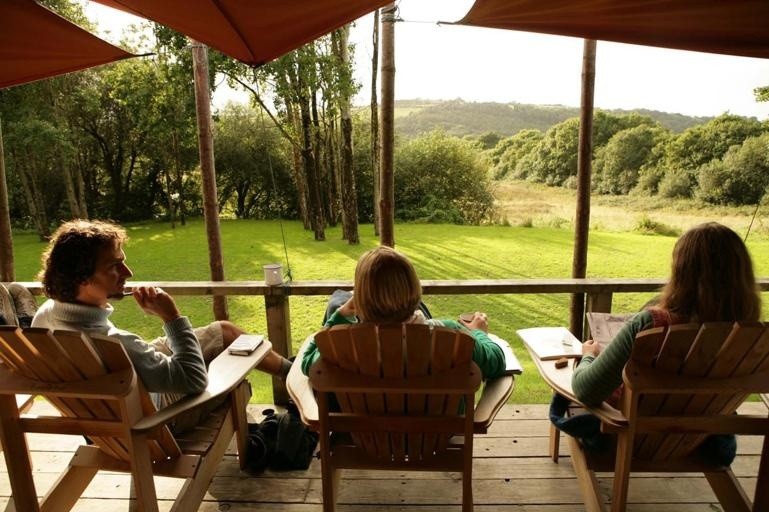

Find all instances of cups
(263, 264), (284, 286)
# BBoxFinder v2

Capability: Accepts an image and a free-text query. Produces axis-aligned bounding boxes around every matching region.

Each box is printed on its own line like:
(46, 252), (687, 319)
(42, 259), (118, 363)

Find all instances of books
(228, 334), (265, 357)
(515, 326), (584, 361)
(488, 339), (523, 377)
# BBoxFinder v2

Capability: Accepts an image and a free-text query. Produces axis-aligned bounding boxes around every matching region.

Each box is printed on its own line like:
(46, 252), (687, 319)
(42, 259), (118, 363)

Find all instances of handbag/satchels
(243, 423), (269, 476)
(259, 409), (318, 473)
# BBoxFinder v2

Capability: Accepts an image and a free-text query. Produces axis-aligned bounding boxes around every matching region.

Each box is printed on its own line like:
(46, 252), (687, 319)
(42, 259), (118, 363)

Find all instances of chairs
(284, 323), (517, 512)
(516, 322), (769, 510)
(0, 323), (272, 512)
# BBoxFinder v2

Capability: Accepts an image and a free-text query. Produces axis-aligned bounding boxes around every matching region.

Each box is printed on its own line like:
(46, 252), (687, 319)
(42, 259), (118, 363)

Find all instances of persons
(299, 245), (507, 444)
(0, 281), (39, 328)
(30, 215), (295, 438)
(571, 221), (761, 413)
